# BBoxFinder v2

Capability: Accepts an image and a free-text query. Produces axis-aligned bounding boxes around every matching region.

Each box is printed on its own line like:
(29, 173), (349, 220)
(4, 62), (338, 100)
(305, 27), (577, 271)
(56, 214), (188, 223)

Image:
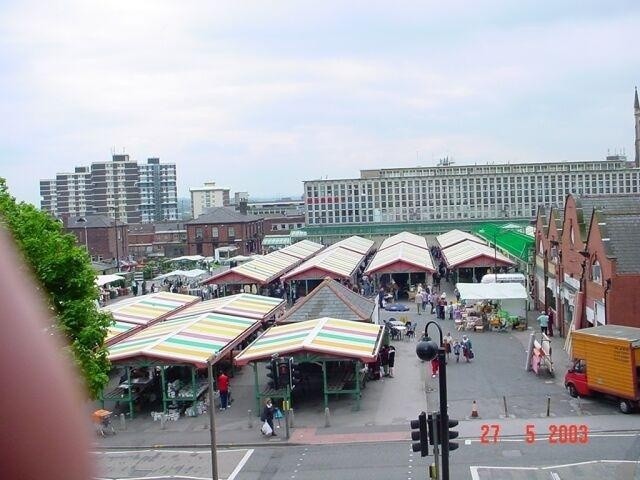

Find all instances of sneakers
(385, 374), (393, 378)
(220, 405), (231, 410)
(272, 431), (277, 436)
(432, 371), (438, 377)
(457, 358), (471, 363)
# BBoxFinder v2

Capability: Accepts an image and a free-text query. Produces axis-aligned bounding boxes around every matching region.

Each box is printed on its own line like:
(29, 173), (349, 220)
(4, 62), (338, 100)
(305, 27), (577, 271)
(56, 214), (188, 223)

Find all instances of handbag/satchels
(275, 409), (283, 418)
(262, 422), (272, 435)
(469, 351), (474, 358)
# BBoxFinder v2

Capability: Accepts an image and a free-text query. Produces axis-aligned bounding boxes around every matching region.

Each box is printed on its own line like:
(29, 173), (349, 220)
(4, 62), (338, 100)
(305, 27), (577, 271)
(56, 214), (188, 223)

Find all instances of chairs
(383, 318), (417, 340)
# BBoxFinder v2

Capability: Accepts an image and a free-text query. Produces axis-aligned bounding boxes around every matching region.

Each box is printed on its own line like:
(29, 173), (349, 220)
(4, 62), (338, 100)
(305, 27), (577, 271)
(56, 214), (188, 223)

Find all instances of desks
(256, 361), (368, 416)
(153, 286), (161, 293)
(383, 293), (395, 302)
(102, 353), (232, 420)
(98, 287), (121, 307)
(452, 304), (526, 333)
(406, 290), (416, 301)
(214, 278), (284, 298)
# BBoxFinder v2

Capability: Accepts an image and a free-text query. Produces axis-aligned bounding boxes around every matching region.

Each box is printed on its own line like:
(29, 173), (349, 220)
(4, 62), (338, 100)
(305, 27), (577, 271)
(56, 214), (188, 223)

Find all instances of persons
(132, 277), (183, 298)
(260, 397), (278, 438)
(216, 369), (230, 410)
(440, 331), (472, 363)
(378, 287), (386, 309)
(536, 311), (550, 335)
(431, 354), (440, 378)
(546, 308), (555, 337)
(379, 345), (390, 377)
(414, 283), (454, 321)
(430, 246), (449, 284)
(386, 346), (396, 378)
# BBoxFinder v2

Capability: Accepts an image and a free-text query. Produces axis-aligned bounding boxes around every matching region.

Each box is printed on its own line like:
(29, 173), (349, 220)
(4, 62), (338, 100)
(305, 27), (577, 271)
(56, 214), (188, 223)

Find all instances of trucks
(564, 324), (640, 413)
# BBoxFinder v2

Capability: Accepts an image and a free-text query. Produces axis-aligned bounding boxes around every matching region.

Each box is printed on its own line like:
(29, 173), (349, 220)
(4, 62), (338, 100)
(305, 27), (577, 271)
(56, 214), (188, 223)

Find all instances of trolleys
(91, 409), (115, 437)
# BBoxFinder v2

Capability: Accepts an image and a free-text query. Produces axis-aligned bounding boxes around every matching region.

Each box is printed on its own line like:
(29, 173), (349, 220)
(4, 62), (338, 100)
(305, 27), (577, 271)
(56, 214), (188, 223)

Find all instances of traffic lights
(448, 418), (459, 450)
(411, 411), (428, 457)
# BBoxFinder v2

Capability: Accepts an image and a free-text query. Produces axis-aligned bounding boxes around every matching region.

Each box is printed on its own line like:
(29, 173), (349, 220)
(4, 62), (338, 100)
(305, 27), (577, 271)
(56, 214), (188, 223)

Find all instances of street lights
(416, 320), (449, 480)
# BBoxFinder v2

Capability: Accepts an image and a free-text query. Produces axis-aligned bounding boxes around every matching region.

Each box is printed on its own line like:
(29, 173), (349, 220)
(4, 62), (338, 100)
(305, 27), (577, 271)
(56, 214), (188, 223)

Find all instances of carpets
(384, 300), (410, 312)
